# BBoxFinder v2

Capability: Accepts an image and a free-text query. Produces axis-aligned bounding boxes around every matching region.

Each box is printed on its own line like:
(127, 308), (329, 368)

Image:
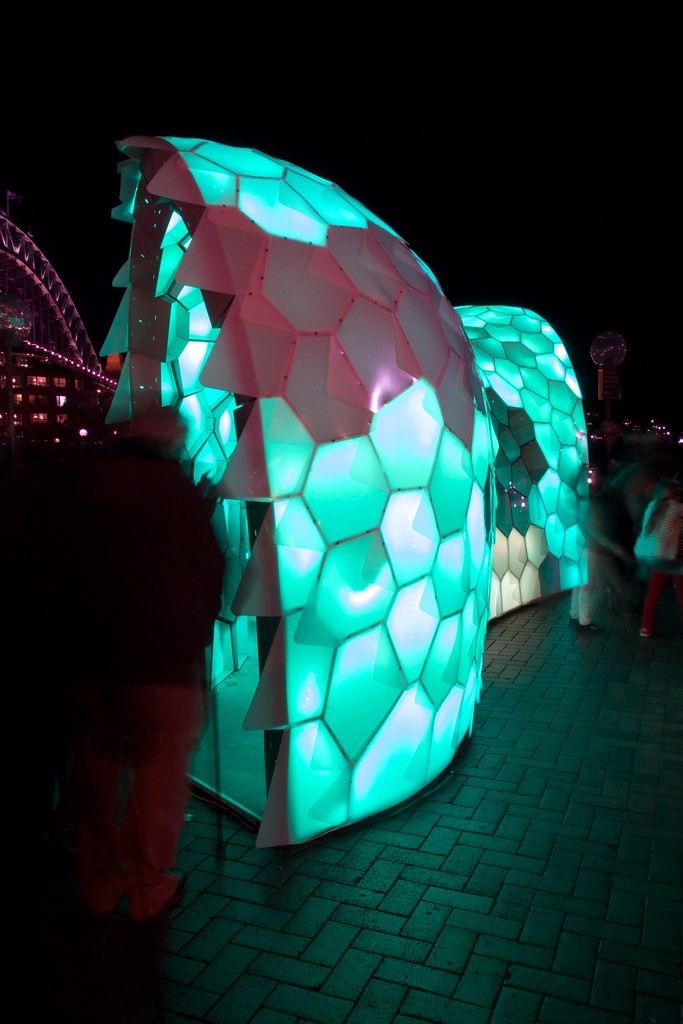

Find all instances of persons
(71, 405), (222, 924)
(570, 444), (683, 637)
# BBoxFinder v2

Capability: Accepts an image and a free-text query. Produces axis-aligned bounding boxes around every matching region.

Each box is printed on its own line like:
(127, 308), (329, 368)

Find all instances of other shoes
(578, 623), (605, 633)
(156, 868), (189, 918)
(639, 626), (652, 637)
